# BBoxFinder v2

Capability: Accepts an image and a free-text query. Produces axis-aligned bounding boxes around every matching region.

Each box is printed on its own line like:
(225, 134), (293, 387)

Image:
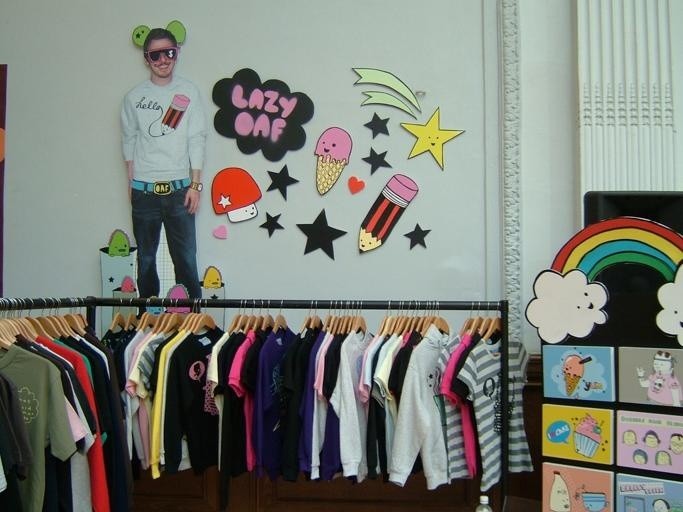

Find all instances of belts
(130, 177), (192, 196)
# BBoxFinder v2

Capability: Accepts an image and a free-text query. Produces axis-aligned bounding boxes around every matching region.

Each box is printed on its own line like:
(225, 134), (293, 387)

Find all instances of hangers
(0, 296), (500, 351)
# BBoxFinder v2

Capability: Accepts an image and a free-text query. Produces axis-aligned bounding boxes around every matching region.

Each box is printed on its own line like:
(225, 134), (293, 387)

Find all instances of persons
(119, 27), (207, 312)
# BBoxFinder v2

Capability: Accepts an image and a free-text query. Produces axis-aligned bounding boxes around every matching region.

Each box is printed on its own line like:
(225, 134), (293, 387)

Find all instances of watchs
(188, 182), (203, 191)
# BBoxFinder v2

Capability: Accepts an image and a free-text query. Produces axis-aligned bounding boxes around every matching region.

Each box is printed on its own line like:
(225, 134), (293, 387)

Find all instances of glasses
(144, 46), (180, 63)
